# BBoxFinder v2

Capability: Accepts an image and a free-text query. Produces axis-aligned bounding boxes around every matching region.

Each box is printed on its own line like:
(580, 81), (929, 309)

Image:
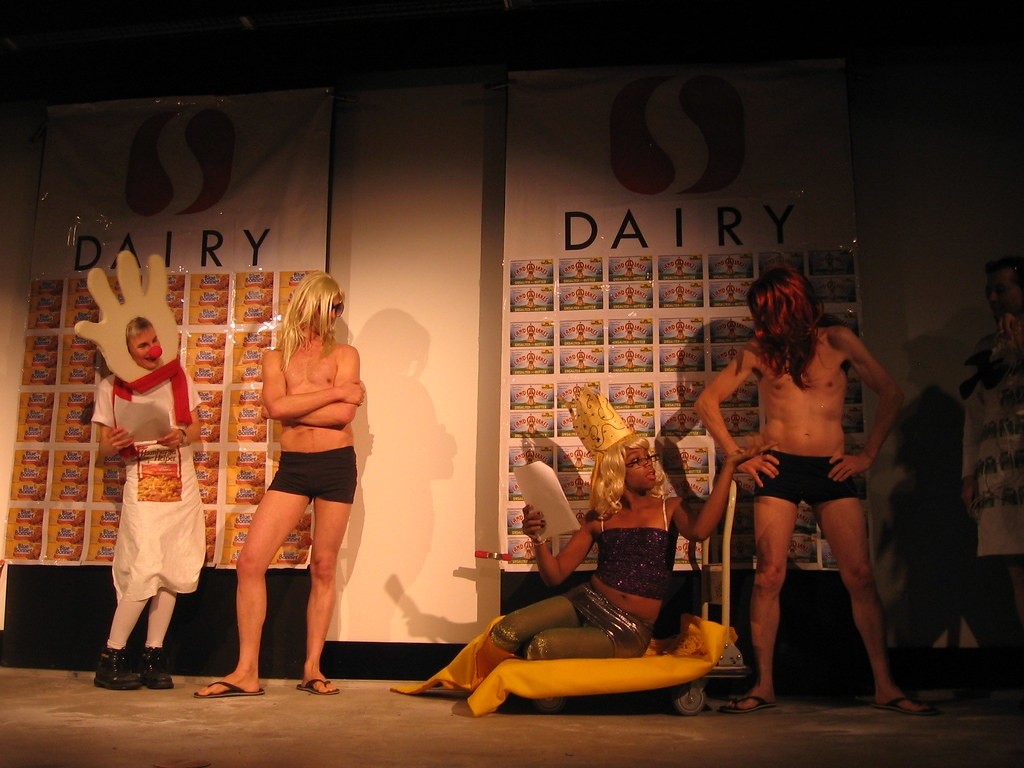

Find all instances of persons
(694, 267), (933, 712)
(425, 389), (779, 718)
(92, 315), (206, 690)
(962, 257), (1024, 625)
(196, 271), (364, 697)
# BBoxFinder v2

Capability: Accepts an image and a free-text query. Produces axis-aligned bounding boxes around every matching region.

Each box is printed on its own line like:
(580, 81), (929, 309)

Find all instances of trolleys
(530, 480), (752, 716)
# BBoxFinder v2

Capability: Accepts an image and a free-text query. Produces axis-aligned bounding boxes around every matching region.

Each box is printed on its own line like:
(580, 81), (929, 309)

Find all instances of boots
(92, 645), (143, 690)
(136, 644), (174, 690)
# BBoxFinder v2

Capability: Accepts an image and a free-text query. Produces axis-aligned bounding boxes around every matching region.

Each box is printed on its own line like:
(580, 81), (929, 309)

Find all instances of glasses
(317, 301), (345, 318)
(624, 453), (661, 471)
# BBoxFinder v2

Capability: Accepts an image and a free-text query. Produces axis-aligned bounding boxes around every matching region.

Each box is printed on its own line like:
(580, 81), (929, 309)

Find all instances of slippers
(717, 696), (781, 713)
(192, 681), (265, 700)
(868, 694), (944, 717)
(297, 678), (341, 695)
